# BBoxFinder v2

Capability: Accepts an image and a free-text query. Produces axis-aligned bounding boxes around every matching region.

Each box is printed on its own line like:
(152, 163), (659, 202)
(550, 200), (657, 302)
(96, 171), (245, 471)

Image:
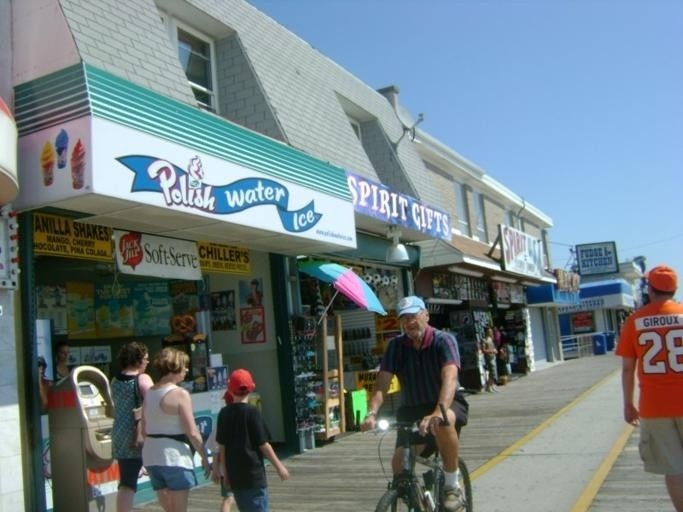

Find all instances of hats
(396, 296), (426, 319)
(645, 265), (678, 292)
(223, 369), (255, 404)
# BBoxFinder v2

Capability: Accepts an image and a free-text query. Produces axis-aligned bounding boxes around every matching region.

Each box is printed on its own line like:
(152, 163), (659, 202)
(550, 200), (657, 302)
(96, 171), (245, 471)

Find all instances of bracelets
(364, 411), (376, 419)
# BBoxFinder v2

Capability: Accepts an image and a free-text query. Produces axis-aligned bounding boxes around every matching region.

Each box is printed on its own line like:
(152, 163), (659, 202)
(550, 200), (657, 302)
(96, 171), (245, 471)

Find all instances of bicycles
(369, 406), (473, 511)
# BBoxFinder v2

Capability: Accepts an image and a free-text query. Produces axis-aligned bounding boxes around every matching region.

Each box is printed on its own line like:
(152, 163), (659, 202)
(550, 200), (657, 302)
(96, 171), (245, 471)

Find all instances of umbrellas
(298, 261), (388, 326)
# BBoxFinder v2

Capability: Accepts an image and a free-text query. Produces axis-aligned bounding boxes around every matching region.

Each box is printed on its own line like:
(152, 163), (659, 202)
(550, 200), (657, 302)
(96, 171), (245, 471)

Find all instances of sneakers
(440, 485), (465, 511)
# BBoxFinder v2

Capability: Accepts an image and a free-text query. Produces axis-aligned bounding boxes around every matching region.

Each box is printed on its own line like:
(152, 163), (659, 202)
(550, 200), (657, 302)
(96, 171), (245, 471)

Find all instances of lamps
(386, 225), (409, 263)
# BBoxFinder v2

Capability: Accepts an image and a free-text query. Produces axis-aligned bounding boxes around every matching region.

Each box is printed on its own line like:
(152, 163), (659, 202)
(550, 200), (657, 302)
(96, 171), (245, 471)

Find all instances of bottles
(422, 486), (435, 512)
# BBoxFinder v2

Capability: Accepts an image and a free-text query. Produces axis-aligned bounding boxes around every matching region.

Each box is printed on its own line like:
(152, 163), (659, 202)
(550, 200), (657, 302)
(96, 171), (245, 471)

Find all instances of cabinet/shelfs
(305, 315), (346, 441)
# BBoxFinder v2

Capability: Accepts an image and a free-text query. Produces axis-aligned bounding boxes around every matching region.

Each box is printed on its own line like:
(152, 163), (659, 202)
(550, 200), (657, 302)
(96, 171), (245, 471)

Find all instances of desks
(355, 371), (402, 414)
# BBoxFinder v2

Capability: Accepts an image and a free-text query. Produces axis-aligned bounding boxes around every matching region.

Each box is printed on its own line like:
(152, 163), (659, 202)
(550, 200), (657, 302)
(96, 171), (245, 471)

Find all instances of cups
(55, 146), (67, 169)
(41, 162), (54, 187)
(70, 165), (86, 190)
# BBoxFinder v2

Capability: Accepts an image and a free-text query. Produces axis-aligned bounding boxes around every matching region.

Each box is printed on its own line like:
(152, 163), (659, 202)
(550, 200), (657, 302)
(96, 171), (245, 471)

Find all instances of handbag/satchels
(133, 406), (147, 447)
(185, 431), (207, 455)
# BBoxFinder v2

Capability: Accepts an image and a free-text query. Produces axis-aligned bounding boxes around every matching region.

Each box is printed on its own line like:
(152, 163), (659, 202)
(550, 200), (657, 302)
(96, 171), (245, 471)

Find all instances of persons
(480, 326), (498, 393)
(55, 344), (72, 382)
(360, 295), (469, 511)
(614, 265), (682, 512)
(215, 368), (289, 512)
(141, 347), (211, 512)
(110, 341), (155, 512)
(246, 279), (263, 307)
(211, 388), (234, 512)
